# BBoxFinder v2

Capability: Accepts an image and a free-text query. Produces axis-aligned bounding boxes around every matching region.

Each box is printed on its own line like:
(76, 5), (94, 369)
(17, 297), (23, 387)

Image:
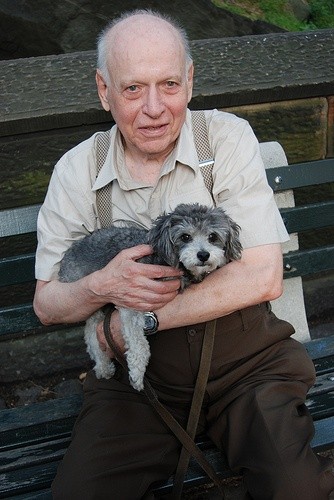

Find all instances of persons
(32, 10), (334, 500)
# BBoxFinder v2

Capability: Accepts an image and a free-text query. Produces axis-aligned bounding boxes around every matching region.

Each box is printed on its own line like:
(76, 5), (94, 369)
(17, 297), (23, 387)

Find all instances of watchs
(140, 310), (158, 335)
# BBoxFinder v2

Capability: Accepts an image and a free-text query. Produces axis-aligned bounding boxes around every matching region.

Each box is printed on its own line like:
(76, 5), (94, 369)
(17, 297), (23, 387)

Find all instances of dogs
(58, 202), (243, 392)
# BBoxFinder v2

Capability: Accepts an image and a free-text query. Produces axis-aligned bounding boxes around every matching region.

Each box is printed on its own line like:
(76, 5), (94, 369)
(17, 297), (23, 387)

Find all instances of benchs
(0, 156), (334, 500)
